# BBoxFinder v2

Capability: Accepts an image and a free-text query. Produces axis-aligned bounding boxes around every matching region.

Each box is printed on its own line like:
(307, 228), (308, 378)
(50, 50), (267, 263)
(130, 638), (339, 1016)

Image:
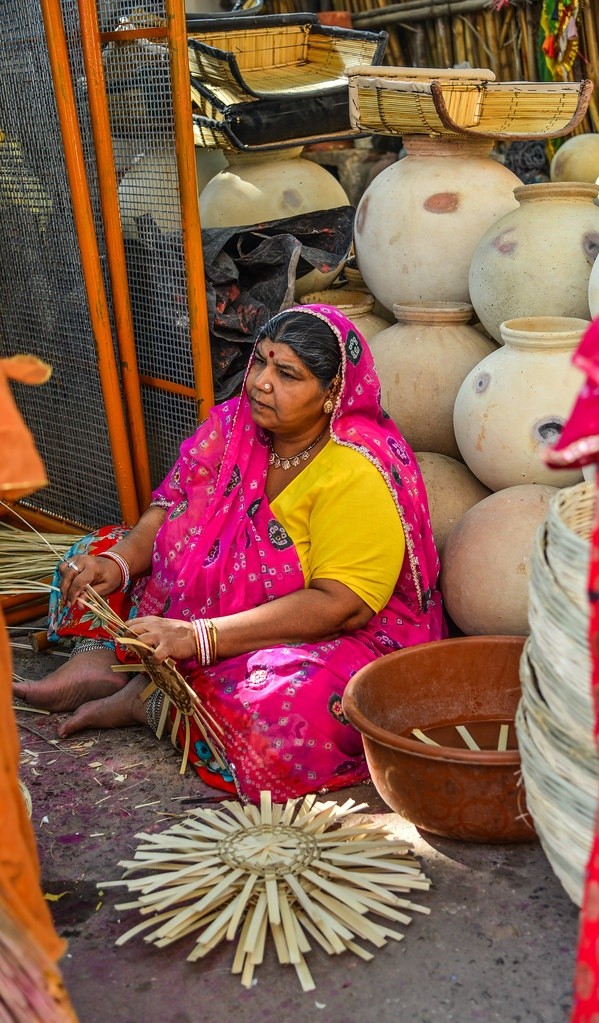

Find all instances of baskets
(517, 480), (599, 910)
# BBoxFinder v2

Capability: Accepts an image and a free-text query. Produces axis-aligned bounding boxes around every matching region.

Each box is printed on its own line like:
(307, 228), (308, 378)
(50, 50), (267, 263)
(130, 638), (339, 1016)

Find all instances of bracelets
(96, 552), (132, 592)
(192, 618), (218, 666)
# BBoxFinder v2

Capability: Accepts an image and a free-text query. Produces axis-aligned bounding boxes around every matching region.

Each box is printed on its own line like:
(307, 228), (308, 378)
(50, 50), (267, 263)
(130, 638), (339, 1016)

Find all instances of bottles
(118, 144), (351, 241)
(353, 135), (523, 314)
(368, 300), (499, 466)
(468, 181), (599, 345)
(299, 290), (392, 343)
(452, 316), (592, 493)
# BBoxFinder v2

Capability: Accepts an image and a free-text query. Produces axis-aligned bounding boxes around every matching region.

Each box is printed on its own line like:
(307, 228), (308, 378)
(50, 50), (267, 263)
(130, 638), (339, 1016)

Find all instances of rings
(68, 561), (80, 574)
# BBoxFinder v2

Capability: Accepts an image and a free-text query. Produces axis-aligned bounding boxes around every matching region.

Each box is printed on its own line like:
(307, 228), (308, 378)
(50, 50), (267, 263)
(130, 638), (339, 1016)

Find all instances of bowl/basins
(341, 634), (537, 846)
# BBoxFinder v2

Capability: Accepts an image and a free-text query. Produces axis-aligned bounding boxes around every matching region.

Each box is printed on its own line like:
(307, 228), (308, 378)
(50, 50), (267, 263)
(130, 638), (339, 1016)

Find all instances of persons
(13, 303), (451, 804)
(0, 356), (74, 1023)
